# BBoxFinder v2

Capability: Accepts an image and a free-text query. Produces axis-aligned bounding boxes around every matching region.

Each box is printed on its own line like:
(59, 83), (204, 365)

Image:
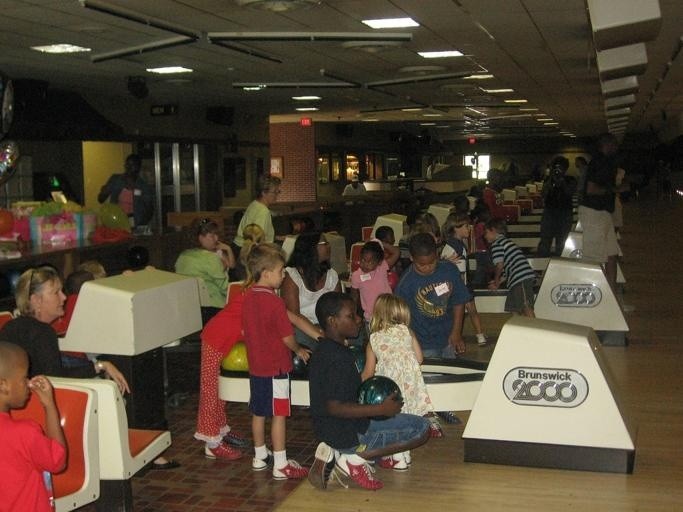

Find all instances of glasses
(318, 241), (328, 245)
(266, 189), (281, 193)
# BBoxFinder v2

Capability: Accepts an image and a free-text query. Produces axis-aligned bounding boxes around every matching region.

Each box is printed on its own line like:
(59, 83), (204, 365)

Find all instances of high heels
(152, 459), (180, 469)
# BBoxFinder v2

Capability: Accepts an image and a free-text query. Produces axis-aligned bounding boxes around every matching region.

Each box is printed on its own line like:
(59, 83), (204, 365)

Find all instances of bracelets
(97, 360), (105, 372)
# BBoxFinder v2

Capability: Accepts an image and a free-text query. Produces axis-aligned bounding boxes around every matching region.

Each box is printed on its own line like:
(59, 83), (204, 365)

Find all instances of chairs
(44, 374), (171, 512)
(5, 383), (101, 512)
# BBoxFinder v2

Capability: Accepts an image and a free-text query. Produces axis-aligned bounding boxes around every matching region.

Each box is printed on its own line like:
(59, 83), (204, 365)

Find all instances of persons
(484, 213), (538, 320)
(0, 342), (68, 512)
(194, 241), (349, 460)
(235, 223), (265, 280)
(287, 216), (314, 266)
(350, 238), (399, 340)
(343, 175), (366, 196)
(0, 266), (182, 468)
(376, 226), (403, 274)
(408, 224), (462, 264)
(309, 291), (432, 493)
(577, 132), (637, 311)
(215, 241), (235, 269)
(440, 212), (487, 346)
(453, 194), (469, 214)
(241, 244), (312, 480)
(175, 216), (235, 342)
(359, 292), (434, 473)
(473, 202), (492, 286)
(483, 168), (506, 216)
(234, 172), (282, 260)
(279, 230), (342, 336)
(612, 167), (625, 236)
(536, 156), (577, 258)
(97, 153), (155, 228)
(393, 233), (472, 362)
(113, 265), (187, 408)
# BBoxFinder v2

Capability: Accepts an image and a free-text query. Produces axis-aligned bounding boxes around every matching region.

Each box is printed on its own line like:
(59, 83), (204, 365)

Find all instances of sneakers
(378, 452), (407, 470)
(403, 451), (411, 464)
(440, 410), (459, 424)
(426, 416), (441, 437)
(253, 453), (274, 472)
(477, 332), (487, 346)
(205, 442), (241, 460)
(619, 298), (636, 312)
(336, 453), (384, 489)
(222, 430), (251, 448)
(308, 441), (336, 490)
(272, 459), (309, 481)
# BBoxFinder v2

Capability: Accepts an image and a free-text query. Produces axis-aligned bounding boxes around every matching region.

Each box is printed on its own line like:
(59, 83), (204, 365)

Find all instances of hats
(351, 175), (360, 181)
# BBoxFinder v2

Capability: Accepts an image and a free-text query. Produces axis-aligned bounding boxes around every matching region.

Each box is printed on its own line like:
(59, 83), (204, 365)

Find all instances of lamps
(483, 88), (513, 93)
(417, 51), (464, 58)
(147, 67), (193, 74)
(244, 86), (261, 91)
(504, 98), (526, 103)
(463, 74), (492, 80)
(362, 17), (420, 29)
(292, 94), (323, 99)
(30, 43), (94, 53)
(297, 107), (317, 110)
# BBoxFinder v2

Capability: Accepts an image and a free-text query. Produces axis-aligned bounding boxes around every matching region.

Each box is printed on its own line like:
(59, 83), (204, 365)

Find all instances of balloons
(101, 203), (132, 233)
(0, 209), (13, 236)
(0, 139), (19, 184)
